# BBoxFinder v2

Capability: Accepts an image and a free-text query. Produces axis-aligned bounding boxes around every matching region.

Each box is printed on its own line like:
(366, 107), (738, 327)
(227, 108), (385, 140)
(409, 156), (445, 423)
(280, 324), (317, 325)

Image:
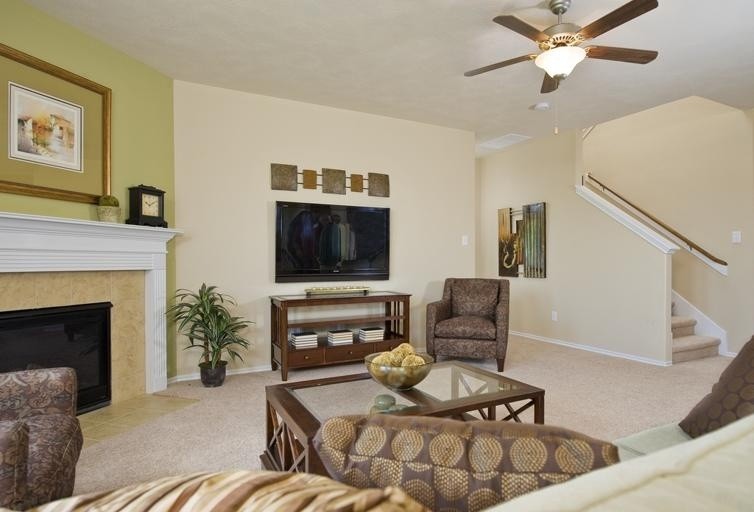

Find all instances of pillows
(449, 278), (500, 321)
(312, 413), (618, 512)
(679, 335), (754, 438)
(27, 471), (433, 512)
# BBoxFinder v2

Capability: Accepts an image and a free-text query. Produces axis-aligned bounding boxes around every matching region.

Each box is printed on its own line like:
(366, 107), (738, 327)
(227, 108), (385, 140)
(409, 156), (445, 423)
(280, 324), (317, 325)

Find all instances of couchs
(482, 423), (754, 512)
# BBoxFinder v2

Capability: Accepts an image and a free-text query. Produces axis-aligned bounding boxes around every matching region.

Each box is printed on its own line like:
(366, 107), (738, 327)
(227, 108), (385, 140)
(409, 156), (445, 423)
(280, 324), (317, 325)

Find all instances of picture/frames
(0, 44), (112, 204)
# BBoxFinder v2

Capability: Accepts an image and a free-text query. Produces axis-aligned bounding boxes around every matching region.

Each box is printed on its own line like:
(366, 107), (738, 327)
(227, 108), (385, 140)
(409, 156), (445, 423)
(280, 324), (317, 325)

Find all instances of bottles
(369, 394), (395, 417)
(389, 404), (409, 412)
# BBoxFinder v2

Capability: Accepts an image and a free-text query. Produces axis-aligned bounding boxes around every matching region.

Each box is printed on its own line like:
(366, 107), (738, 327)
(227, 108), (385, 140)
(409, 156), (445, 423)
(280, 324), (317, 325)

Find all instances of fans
(464, 0), (658, 94)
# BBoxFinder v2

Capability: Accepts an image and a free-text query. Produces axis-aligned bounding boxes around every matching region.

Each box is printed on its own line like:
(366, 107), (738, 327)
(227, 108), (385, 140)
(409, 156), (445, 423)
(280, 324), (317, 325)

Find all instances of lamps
(533, 43), (586, 80)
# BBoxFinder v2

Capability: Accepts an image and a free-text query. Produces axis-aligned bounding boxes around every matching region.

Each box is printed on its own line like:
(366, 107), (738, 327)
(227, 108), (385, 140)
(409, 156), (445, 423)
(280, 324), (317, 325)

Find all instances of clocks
(126, 184), (169, 228)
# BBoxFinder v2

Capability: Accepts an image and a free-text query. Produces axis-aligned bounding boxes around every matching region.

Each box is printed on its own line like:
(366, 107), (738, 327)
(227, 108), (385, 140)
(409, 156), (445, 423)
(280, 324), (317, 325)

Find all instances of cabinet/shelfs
(269, 291), (412, 381)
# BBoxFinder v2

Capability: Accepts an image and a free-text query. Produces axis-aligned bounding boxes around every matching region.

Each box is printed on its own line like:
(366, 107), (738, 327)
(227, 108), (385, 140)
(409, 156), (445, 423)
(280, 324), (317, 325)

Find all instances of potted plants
(165, 282), (256, 387)
(96, 195), (120, 222)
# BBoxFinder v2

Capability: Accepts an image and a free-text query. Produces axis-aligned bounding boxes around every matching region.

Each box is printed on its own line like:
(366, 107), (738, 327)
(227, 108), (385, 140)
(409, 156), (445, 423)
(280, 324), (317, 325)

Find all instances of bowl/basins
(363, 352), (435, 392)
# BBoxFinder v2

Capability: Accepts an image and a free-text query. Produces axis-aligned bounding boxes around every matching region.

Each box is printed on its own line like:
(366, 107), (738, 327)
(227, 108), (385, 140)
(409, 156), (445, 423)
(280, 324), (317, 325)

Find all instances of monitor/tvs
(275, 200), (390, 281)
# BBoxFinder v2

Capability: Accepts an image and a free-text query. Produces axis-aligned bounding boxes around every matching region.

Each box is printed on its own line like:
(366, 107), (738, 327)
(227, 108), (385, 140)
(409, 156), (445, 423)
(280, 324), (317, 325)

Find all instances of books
(291, 332), (318, 349)
(359, 328), (384, 342)
(327, 330), (353, 347)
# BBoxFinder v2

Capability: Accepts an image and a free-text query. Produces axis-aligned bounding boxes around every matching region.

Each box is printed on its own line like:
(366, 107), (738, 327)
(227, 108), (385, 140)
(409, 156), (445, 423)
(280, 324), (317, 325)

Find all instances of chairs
(0, 367), (83, 512)
(426, 278), (510, 372)
